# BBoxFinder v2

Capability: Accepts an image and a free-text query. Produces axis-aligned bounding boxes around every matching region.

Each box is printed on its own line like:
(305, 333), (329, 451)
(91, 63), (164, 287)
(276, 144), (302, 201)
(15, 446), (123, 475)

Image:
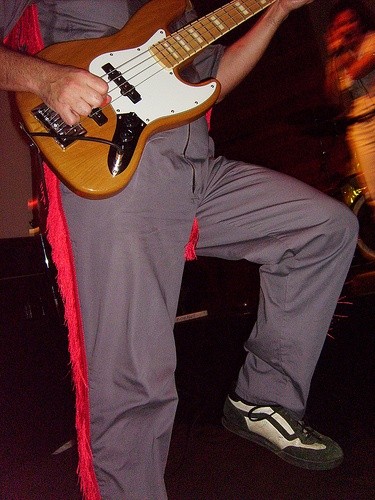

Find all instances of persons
(324, 3), (374, 208)
(0, 0), (359, 500)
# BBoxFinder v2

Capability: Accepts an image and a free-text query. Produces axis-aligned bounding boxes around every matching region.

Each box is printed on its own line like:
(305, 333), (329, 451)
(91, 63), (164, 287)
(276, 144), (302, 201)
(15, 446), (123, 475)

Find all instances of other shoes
(222, 390), (344, 469)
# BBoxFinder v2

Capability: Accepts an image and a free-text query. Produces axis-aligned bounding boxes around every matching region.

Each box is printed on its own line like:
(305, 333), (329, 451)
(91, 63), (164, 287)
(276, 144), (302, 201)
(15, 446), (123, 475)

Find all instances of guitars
(13, 0), (280, 199)
